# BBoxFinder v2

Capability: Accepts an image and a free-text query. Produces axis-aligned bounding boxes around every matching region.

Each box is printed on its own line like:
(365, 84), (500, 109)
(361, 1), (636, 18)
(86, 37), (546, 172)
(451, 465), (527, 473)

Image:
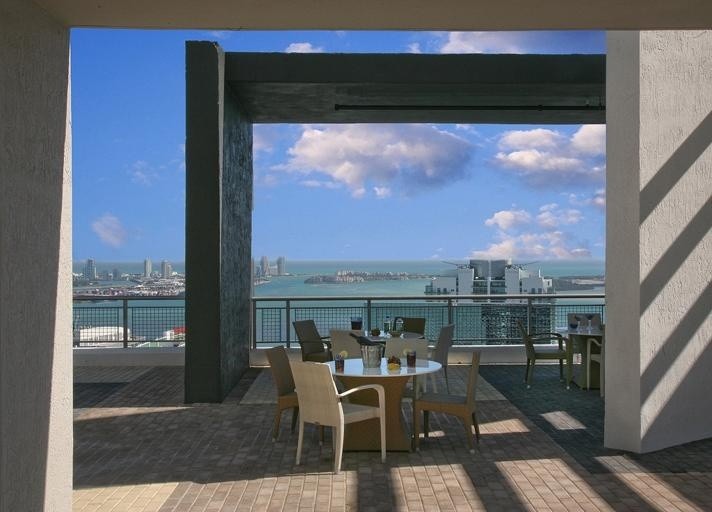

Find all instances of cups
(350, 317), (363, 330)
(407, 350), (416, 367)
(334, 353), (344, 371)
(570, 319), (600, 335)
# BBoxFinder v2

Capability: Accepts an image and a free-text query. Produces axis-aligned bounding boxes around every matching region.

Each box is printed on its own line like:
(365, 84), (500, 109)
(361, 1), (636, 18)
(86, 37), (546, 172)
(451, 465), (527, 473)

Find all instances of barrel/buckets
(360, 343), (381, 368)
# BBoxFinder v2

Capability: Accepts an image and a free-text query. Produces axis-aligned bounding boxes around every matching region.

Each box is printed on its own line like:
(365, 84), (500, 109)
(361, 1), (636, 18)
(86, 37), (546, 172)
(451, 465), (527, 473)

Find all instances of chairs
(516, 319), (569, 389)
(265, 345), (324, 446)
(413, 350), (480, 455)
(292, 319), (333, 362)
(393, 316), (425, 335)
(567, 312), (601, 331)
(328, 329), (365, 360)
(428, 325), (455, 394)
(586, 327), (605, 398)
(289, 358), (386, 474)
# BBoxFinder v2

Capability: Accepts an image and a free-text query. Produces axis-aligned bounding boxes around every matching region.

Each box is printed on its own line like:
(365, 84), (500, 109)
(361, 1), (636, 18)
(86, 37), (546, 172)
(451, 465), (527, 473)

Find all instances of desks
(567, 331), (602, 389)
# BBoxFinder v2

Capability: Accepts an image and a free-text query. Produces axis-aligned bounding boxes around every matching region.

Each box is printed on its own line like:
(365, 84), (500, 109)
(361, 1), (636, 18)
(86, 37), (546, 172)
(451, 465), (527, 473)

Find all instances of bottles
(384, 315), (392, 338)
(395, 317), (404, 338)
(349, 331), (374, 345)
